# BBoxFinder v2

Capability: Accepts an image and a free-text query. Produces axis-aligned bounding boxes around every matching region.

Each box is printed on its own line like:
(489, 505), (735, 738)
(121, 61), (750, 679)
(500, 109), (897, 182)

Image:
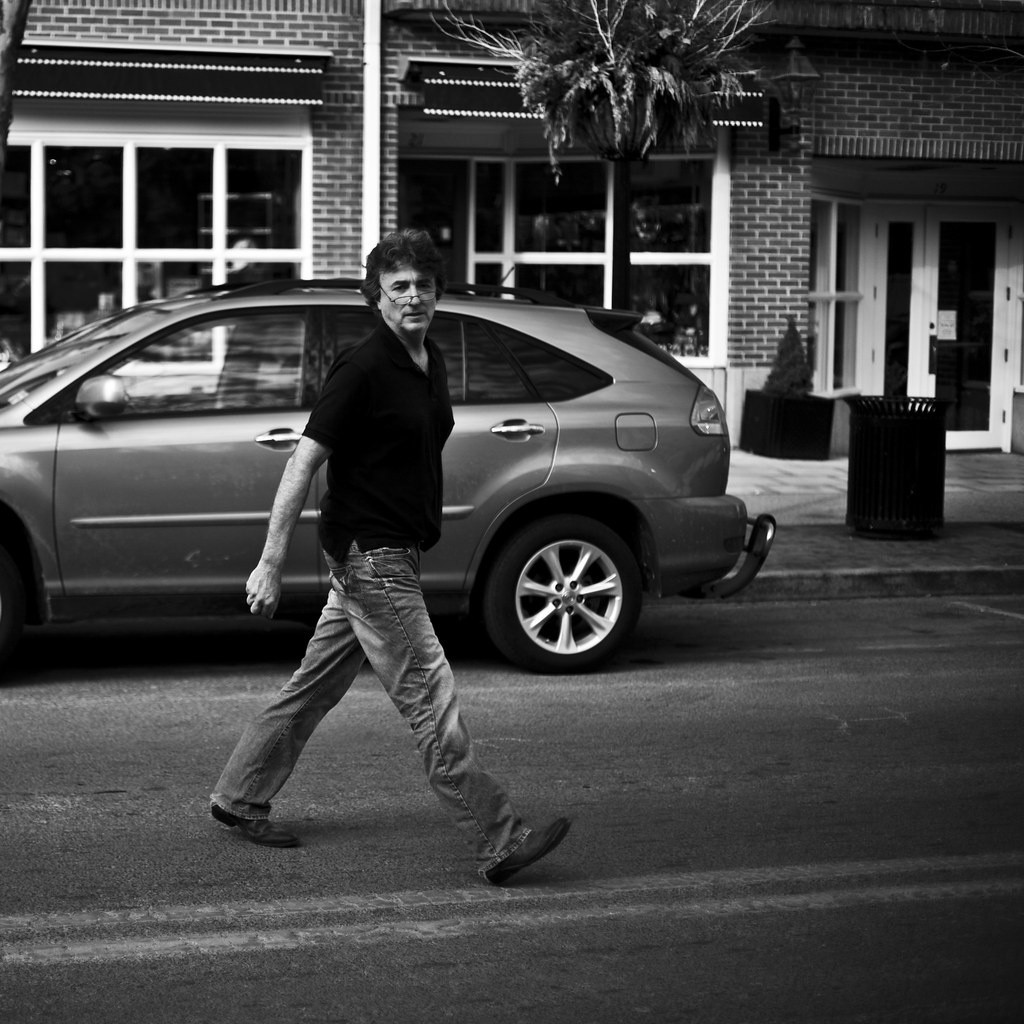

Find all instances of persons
(208, 226), (570, 885)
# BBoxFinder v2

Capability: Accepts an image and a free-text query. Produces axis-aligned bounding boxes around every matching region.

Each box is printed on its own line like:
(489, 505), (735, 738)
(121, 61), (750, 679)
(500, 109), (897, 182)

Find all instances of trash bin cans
(837, 393), (956, 538)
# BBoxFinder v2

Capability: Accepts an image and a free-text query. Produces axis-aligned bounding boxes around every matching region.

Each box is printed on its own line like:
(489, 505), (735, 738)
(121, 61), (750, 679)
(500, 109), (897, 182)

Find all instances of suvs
(0, 278), (780, 675)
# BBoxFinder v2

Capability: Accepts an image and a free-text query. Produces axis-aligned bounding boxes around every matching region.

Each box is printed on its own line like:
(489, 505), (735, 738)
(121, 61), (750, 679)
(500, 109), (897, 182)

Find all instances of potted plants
(738, 314), (835, 460)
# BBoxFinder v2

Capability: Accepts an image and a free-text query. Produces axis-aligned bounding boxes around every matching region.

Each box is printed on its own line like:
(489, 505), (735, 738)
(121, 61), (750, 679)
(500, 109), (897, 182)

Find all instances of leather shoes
(485, 816), (571, 884)
(212, 805), (300, 847)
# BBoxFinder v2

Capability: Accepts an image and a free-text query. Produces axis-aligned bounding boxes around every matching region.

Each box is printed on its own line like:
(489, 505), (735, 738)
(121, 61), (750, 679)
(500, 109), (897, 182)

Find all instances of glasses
(378, 284), (439, 305)
